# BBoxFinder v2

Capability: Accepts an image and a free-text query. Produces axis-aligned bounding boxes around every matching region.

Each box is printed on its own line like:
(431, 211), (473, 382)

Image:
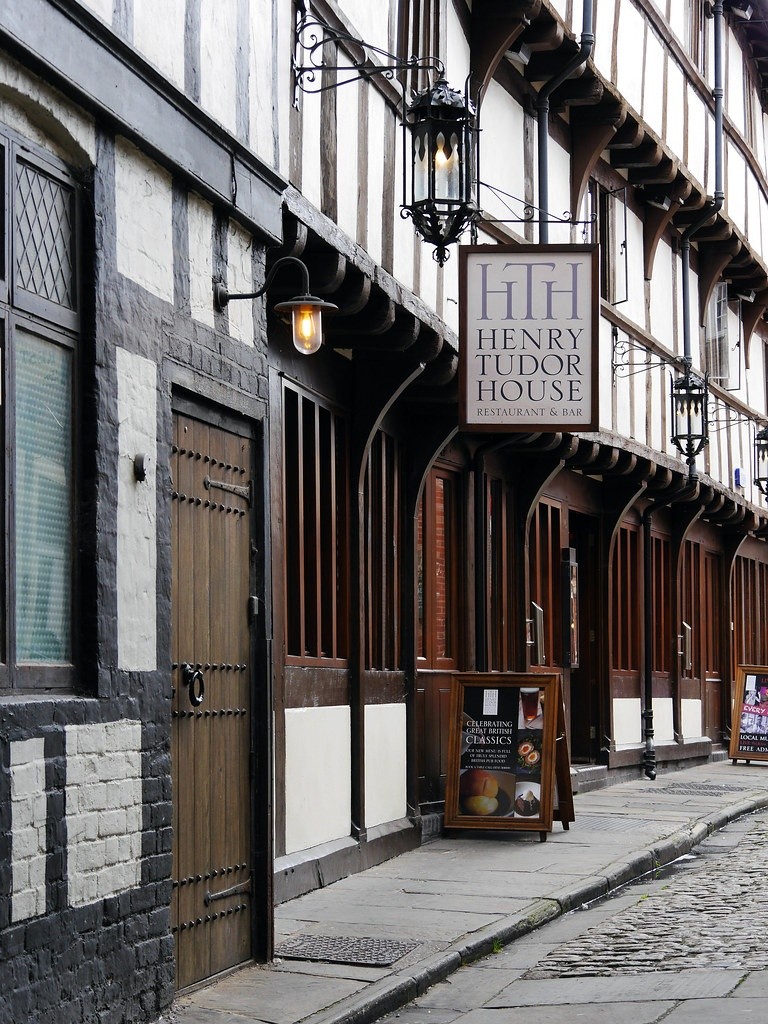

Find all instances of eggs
(521, 744), (537, 762)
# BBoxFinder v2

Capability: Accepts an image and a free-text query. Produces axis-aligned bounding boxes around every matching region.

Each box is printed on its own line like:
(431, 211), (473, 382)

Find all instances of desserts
(514, 789), (538, 816)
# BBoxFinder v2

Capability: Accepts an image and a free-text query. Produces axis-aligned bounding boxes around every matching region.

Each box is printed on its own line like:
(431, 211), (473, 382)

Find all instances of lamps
(706, 401), (768, 501)
(210, 255), (339, 354)
(608, 326), (711, 473)
(292, 10), (474, 266)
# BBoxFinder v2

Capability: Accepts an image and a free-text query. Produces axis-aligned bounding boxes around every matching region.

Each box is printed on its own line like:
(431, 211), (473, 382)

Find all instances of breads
(464, 770), (499, 816)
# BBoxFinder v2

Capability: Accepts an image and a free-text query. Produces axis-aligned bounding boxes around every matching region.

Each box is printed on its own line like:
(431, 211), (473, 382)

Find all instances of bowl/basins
(460, 769), (516, 817)
(513, 782), (540, 817)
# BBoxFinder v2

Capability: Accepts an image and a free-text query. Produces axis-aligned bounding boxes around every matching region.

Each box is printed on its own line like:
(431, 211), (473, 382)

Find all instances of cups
(520, 688), (540, 721)
